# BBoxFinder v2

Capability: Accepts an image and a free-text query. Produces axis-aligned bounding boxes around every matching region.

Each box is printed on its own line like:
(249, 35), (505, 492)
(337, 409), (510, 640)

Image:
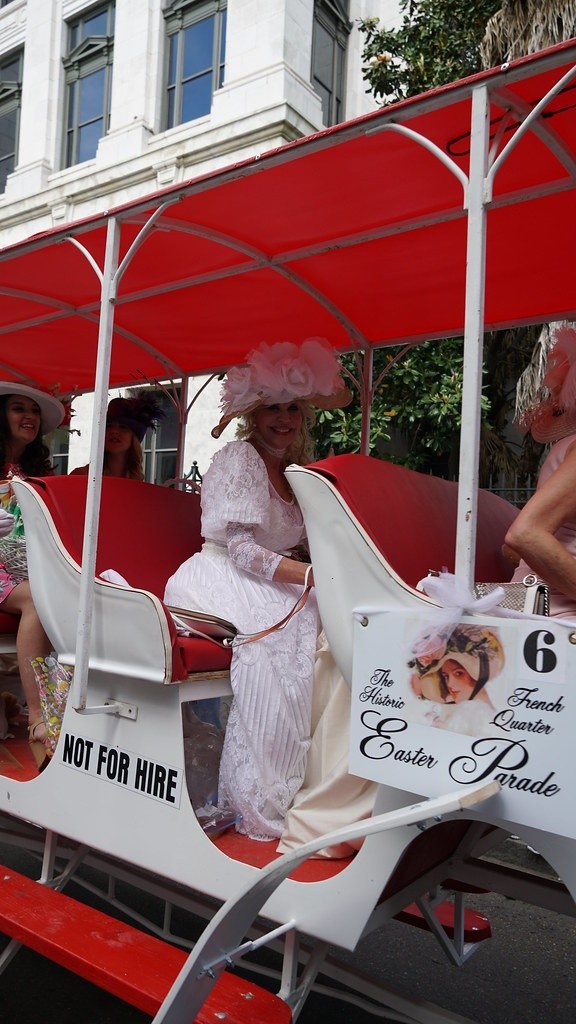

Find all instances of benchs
(283, 451), (576, 883)
(0, 473), (234, 819)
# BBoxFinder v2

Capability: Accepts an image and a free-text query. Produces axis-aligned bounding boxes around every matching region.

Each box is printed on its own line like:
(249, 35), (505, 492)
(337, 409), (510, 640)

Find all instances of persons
(1, 378), (98, 774)
(72, 394), (167, 480)
(403, 651), (501, 738)
(498, 320), (576, 629)
(160, 342), (378, 863)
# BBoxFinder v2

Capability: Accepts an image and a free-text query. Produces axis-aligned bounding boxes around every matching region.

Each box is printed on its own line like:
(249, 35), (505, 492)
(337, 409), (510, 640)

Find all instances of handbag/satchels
(165, 566), (313, 648)
(0, 500), (29, 581)
(415, 567), (550, 616)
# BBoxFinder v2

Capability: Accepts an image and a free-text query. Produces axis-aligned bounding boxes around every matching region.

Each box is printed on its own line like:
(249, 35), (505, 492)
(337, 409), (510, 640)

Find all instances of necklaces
(252, 431), (287, 459)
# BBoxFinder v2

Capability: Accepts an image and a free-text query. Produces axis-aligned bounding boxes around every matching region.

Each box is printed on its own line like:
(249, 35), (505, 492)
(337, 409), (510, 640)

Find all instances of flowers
(219, 336), (342, 419)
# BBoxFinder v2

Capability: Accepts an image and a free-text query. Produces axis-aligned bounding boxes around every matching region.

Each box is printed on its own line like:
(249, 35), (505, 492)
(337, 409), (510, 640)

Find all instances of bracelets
(303, 565), (312, 589)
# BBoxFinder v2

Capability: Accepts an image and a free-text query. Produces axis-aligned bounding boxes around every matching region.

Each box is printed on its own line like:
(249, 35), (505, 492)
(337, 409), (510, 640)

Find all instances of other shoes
(27, 720), (47, 773)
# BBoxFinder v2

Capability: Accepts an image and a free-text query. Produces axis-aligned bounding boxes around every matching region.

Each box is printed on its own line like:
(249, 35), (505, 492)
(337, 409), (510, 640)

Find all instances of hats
(512, 320), (576, 444)
(211, 340), (353, 439)
(0, 381), (66, 436)
(107, 390), (168, 433)
